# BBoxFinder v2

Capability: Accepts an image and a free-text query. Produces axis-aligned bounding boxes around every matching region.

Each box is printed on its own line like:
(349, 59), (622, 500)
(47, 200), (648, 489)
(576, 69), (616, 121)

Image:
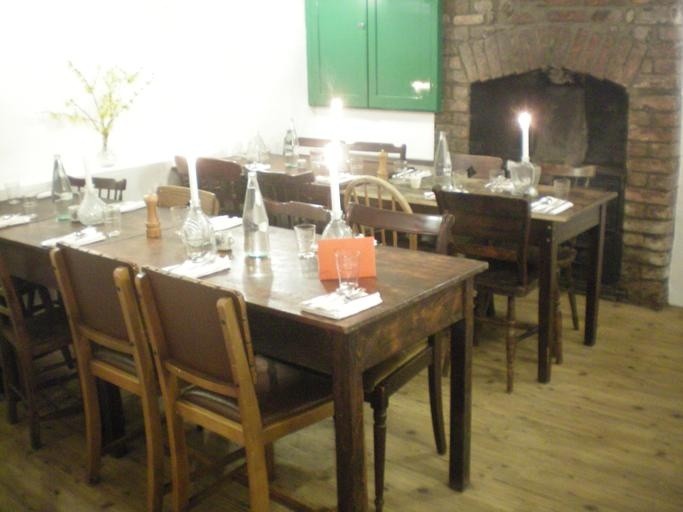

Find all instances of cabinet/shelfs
(305, 0), (442, 112)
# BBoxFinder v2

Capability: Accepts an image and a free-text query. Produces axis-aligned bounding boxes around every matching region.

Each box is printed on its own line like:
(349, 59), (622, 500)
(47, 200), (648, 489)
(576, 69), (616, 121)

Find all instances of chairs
(344, 201), (453, 511)
(156, 186), (219, 216)
(344, 176), (417, 250)
(450, 152), (505, 179)
(431, 185), (564, 394)
(92, 177), (128, 203)
(0, 237), (84, 449)
(341, 142), (407, 165)
(133, 265), (335, 512)
(256, 171), (316, 201)
(50, 241), (192, 512)
(197, 158), (249, 218)
(67, 176), (87, 192)
(294, 137), (331, 159)
(264, 200), (332, 234)
(530, 162), (597, 330)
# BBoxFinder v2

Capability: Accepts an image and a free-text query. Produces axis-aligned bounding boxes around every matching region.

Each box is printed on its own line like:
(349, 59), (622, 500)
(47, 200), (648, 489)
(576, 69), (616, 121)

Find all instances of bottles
(242, 169), (272, 261)
(282, 117), (299, 168)
(434, 131), (451, 191)
(50, 154), (76, 205)
(182, 199), (215, 247)
(510, 157), (541, 197)
(322, 210), (353, 238)
(78, 185), (109, 226)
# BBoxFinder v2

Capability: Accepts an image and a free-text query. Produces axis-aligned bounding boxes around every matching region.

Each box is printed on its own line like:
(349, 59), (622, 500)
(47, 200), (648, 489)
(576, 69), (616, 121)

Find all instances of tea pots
(53, 191), (81, 221)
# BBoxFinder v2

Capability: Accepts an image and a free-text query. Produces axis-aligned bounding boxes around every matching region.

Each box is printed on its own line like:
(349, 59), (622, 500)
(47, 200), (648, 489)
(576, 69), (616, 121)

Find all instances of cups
(67, 204), (81, 223)
(393, 159), (409, 184)
(349, 151), (363, 174)
(298, 153), (310, 169)
(451, 170), (469, 192)
(553, 178), (571, 201)
(255, 144), (274, 169)
(214, 230), (235, 251)
(24, 184), (37, 211)
(411, 170), (421, 189)
(311, 144), (324, 161)
(511, 175), (531, 196)
(5, 180), (21, 205)
(489, 168), (505, 193)
(336, 247), (361, 295)
(102, 206), (121, 239)
(170, 206), (191, 233)
(293, 223), (317, 262)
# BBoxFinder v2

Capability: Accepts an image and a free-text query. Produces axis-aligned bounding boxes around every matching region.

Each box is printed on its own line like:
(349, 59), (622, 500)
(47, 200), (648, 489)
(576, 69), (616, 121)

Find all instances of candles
(75, 143), (104, 185)
(170, 128), (208, 200)
(518, 110), (531, 162)
(329, 97), (344, 214)
